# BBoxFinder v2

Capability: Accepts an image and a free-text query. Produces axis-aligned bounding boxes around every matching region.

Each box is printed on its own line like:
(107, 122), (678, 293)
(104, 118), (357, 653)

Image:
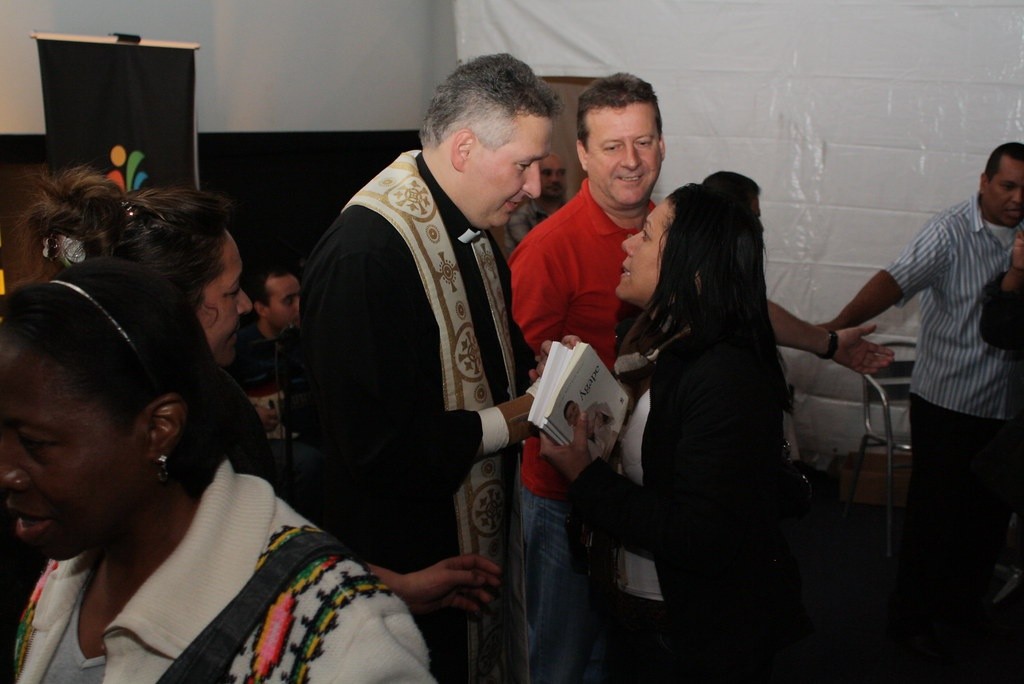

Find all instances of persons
(509, 72), (895, 684)
(540, 172), (792, 684)
(298, 53), (580, 684)
(0, 169), (502, 684)
(564, 400), (619, 461)
(817, 142), (1024, 656)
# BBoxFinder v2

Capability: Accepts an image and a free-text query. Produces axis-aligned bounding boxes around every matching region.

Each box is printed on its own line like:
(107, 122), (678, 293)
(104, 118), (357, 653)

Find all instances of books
(528, 341), (628, 463)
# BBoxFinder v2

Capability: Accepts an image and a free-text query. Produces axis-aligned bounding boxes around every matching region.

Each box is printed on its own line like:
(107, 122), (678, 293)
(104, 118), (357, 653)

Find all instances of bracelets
(818, 328), (838, 359)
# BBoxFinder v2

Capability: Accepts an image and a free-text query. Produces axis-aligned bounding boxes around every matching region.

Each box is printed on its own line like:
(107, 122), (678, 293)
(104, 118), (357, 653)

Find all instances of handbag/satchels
(770, 439), (812, 516)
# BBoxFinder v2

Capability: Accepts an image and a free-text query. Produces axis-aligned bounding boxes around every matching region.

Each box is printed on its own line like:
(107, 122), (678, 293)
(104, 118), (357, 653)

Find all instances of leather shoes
(887, 601), (954, 666)
(928, 591), (1018, 640)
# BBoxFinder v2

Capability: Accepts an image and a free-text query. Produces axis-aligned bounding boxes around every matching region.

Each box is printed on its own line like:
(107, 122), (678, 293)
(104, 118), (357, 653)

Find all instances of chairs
(843, 340), (916, 558)
